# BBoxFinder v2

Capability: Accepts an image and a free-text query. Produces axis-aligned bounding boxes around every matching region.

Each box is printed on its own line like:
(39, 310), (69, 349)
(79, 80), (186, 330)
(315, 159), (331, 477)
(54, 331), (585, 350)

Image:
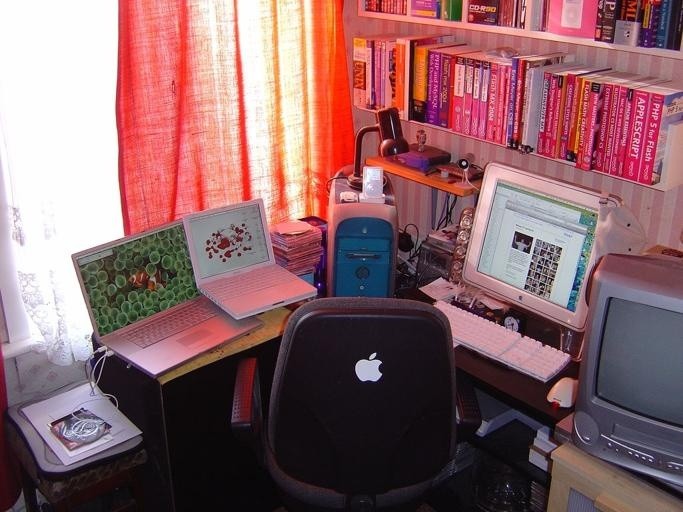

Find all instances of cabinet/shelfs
(546, 441), (683, 512)
(356, 1), (683, 193)
(92, 308), (291, 512)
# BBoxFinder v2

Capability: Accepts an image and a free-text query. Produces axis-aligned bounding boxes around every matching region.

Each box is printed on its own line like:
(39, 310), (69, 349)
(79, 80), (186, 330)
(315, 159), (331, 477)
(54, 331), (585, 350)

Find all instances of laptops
(183, 197), (318, 320)
(71, 218), (264, 379)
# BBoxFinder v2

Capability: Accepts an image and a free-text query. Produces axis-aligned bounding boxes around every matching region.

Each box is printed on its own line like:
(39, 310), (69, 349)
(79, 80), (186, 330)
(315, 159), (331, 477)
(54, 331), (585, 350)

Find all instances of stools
(4, 417), (157, 511)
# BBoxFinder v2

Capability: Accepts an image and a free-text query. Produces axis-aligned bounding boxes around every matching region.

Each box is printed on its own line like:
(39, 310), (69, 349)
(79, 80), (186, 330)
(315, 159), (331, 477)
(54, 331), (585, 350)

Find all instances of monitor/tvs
(462, 160), (651, 333)
(571, 252), (683, 496)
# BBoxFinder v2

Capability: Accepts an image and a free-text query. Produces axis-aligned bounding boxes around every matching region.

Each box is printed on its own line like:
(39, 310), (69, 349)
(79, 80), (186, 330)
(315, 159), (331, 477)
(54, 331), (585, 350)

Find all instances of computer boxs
(327, 165), (399, 297)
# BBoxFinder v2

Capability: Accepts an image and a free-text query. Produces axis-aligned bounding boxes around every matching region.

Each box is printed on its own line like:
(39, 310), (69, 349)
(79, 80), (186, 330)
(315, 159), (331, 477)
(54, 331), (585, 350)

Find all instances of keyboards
(433, 300), (572, 383)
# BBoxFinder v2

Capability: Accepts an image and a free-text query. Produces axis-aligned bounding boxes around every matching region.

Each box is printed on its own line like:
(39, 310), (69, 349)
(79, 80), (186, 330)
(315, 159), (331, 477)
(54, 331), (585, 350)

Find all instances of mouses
(546, 376), (578, 409)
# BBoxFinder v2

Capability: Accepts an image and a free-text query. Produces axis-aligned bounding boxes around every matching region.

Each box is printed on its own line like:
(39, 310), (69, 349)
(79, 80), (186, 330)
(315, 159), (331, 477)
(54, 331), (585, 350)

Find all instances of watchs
(496, 315), (523, 333)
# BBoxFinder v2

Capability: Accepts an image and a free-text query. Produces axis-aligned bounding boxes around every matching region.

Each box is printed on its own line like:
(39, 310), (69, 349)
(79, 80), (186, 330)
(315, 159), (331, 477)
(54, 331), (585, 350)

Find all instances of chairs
(231, 296), (482, 511)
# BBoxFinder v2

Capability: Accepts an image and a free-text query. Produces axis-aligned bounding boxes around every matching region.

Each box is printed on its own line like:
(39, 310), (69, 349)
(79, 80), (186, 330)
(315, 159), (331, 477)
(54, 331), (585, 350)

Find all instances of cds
(62, 416), (105, 443)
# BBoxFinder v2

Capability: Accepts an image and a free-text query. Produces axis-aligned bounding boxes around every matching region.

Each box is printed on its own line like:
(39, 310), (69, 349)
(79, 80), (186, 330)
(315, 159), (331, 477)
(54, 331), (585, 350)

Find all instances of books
(352, 34), (683, 185)
(365, 0), (683, 51)
(269, 220), (323, 276)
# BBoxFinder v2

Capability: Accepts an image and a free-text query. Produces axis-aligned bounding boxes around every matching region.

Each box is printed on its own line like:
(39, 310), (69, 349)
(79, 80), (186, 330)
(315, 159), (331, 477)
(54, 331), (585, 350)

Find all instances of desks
(391, 277), (580, 512)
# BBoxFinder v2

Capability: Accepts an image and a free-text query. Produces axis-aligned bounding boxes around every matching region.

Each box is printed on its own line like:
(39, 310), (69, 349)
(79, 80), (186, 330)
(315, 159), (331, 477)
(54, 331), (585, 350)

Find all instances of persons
(515, 235), (531, 251)
